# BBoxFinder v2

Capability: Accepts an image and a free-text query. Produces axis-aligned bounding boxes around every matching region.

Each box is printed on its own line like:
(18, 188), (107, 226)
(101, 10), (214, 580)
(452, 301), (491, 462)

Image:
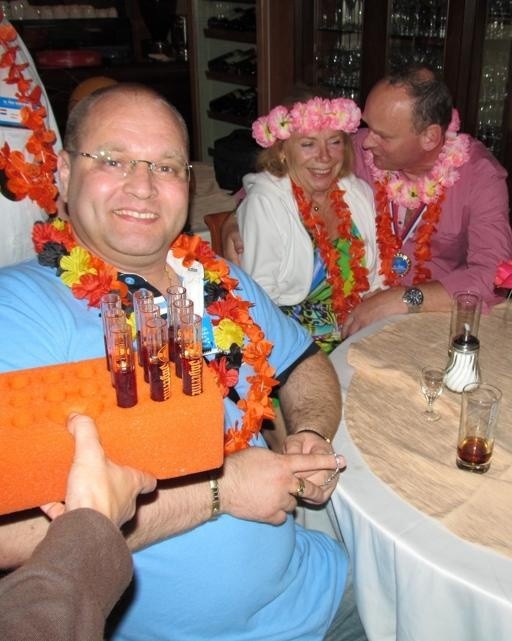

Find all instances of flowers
(371, 181), (443, 291)
(363, 112), (470, 207)
(293, 191), (369, 324)
(30, 218), (279, 461)
(1, 18), (61, 218)
(252, 98), (362, 147)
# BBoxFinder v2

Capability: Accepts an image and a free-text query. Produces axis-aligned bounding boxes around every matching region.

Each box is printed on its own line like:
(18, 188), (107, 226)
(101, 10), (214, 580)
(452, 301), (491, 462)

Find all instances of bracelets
(210, 471), (222, 523)
(303, 449), (343, 488)
(295, 429), (332, 446)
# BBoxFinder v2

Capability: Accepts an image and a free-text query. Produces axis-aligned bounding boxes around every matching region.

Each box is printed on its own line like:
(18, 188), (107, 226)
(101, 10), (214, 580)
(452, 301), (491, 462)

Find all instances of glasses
(66, 149), (193, 186)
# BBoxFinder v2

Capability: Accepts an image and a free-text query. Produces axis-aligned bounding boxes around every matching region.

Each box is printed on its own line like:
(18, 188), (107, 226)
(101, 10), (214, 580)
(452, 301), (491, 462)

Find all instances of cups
(448, 291), (483, 358)
(456, 382), (503, 474)
(420, 366), (448, 422)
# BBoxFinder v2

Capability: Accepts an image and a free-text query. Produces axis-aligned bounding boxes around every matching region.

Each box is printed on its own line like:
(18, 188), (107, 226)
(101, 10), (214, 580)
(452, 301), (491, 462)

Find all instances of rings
(293, 477), (306, 497)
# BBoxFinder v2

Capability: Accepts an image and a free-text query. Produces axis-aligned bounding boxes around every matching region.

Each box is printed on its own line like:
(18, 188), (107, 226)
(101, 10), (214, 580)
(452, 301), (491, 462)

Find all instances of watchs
(402, 282), (424, 315)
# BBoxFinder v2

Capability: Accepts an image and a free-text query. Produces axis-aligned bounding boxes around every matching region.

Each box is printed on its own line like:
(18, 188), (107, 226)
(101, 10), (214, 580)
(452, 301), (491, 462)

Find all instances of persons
(0, 87), (372, 640)
(68, 76), (118, 118)
(0, 413), (157, 641)
(236, 79), (389, 355)
(221, 63), (512, 341)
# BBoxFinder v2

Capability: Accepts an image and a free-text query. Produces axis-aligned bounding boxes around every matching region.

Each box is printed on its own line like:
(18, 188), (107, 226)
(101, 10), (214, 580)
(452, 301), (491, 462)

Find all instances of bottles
(442, 324), (482, 394)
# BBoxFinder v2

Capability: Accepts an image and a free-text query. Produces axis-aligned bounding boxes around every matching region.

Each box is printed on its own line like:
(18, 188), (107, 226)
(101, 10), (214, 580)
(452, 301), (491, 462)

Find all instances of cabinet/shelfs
(363, 0), (449, 133)
(1, 0), (202, 162)
(261, 0), (361, 101)
(449, 1), (512, 162)
(193, 1), (261, 127)
